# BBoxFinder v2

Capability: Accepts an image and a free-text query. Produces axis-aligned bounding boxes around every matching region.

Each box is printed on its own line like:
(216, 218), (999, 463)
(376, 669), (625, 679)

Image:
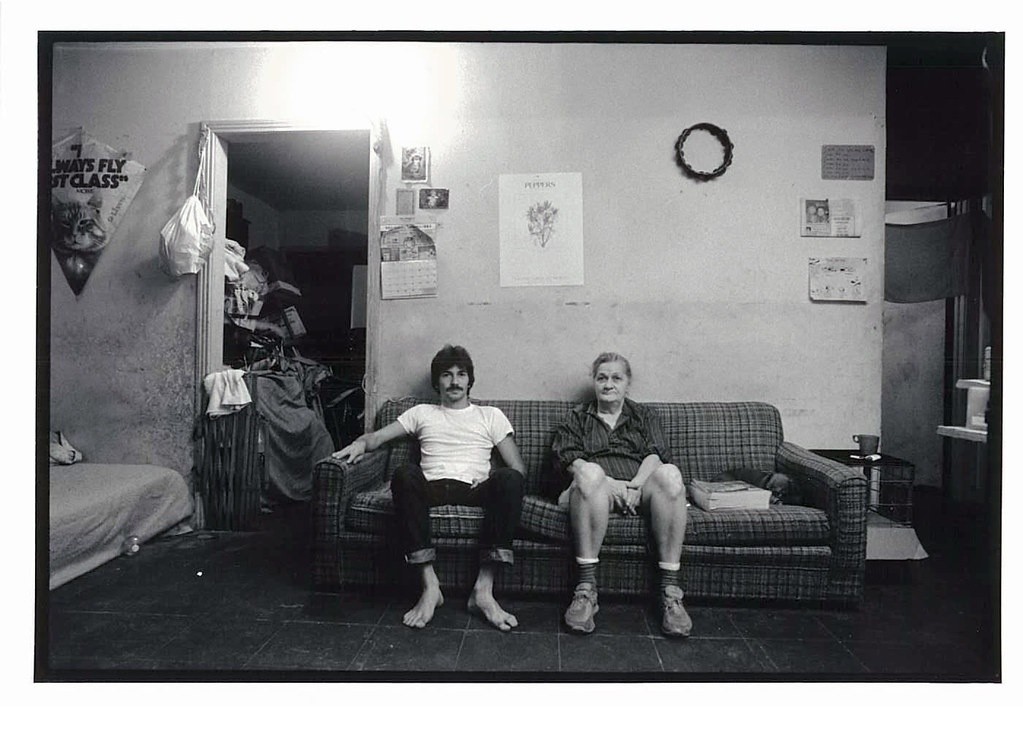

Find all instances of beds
(50, 463), (195, 591)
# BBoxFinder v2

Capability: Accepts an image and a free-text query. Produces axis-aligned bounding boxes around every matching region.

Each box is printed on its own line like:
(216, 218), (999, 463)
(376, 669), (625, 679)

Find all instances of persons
(806, 203), (829, 223)
(330, 343), (527, 633)
(550, 351), (693, 639)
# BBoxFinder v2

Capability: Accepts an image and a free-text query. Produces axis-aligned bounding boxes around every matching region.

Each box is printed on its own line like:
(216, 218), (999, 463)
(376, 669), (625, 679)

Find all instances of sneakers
(564, 582), (694, 639)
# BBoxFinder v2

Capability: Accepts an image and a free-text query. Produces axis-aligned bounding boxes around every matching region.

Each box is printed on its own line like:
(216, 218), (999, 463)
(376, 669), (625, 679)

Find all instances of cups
(853, 434), (879, 456)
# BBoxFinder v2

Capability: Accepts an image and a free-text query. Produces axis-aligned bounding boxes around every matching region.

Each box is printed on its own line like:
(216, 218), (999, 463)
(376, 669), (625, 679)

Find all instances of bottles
(123, 535), (143, 558)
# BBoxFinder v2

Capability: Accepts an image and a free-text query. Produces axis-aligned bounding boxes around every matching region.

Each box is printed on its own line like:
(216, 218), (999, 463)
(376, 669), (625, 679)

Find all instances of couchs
(312, 394), (868, 604)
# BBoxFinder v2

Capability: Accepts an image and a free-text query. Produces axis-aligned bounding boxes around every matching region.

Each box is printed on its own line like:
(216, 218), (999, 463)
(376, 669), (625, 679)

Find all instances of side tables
(809, 450), (914, 528)
